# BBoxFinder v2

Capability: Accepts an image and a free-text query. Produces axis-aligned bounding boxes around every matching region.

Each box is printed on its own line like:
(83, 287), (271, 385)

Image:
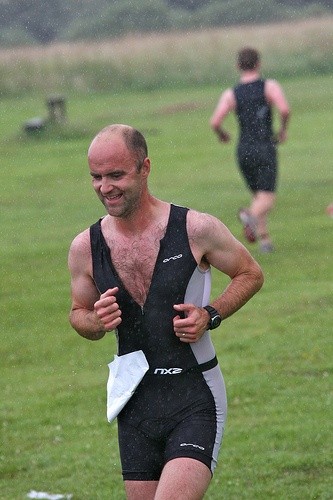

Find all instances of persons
(210, 47), (290, 254)
(67, 123), (263, 500)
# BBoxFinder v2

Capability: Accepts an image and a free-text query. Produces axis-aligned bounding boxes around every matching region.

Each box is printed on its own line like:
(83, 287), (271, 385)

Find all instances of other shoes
(261, 244), (273, 253)
(239, 209), (256, 243)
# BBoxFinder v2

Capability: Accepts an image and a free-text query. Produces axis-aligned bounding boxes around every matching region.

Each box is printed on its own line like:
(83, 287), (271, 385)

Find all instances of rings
(182, 333), (186, 337)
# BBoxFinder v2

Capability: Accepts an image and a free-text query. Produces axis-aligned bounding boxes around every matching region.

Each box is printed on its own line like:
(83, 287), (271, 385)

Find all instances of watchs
(203, 305), (223, 331)
